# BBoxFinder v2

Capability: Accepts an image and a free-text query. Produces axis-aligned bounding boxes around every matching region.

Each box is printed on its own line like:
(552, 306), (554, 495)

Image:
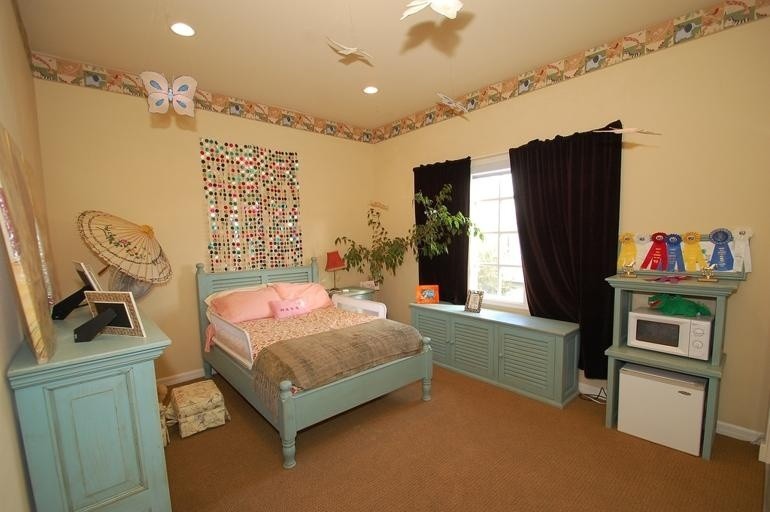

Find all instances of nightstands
(338, 286), (375, 300)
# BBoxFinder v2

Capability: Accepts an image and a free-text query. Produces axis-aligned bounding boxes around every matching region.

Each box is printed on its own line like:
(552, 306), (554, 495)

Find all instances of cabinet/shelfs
(603, 275), (737, 462)
(499, 321), (580, 411)
(409, 305), (499, 387)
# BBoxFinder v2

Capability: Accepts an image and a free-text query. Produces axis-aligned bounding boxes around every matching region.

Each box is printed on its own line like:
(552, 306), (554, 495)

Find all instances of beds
(195, 256), (434, 470)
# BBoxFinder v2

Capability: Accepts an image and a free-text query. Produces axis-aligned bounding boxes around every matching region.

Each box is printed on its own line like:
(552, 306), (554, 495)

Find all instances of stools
(172, 380), (232, 439)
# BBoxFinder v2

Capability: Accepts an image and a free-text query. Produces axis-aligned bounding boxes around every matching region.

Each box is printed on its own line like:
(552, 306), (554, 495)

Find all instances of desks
(8, 306), (172, 512)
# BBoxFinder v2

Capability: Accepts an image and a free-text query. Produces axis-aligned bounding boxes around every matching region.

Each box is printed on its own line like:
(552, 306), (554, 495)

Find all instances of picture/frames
(74, 290), (146, 342)
(52, 260), (102, 320)
(416, 285), (439, 304)
(465, 289), (484, 313)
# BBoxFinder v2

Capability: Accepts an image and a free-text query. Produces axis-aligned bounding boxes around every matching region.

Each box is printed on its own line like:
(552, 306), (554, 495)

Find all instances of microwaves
(626, 305), (715, 362)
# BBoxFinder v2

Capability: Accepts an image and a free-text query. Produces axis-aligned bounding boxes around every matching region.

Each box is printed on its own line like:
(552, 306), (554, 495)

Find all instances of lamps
(325, 251), (346, 290)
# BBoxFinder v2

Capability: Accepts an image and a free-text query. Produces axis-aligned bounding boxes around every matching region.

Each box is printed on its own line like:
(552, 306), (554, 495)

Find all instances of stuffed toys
(646, 292), (712, 318)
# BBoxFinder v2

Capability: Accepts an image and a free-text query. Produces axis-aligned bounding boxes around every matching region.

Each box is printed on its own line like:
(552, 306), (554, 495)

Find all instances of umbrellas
(77, 208), (173, 286)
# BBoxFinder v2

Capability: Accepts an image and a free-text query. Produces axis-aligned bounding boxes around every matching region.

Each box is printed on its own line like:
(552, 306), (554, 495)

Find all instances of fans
(108, 265), (150, 301)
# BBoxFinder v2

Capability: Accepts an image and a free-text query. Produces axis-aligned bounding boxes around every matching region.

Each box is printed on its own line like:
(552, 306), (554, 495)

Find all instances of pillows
(203, 283), (335, 325)
